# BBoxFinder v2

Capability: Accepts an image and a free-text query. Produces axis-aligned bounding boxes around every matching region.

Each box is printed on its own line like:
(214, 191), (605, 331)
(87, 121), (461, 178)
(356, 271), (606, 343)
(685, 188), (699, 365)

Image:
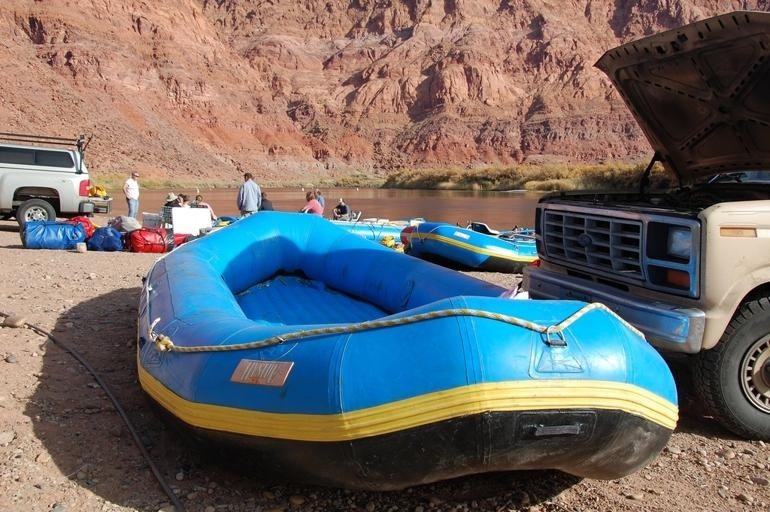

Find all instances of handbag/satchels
(125, 226), (175, 253)
(65, 216), (95, 240)
(108, 215), (142, 233)
(19, 220), (86, 250)
(87, 226), (125, 251)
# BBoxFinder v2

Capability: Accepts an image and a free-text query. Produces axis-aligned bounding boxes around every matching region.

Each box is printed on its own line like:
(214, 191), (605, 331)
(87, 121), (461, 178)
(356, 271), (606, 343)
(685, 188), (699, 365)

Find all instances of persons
(164, 191), (218, 221)
(123, 171), (140, 218)
(299, 189), (351, 221)
(236, 173), (262, 216)
(258, 192), (273, 210)
(511, 224), (519, 232)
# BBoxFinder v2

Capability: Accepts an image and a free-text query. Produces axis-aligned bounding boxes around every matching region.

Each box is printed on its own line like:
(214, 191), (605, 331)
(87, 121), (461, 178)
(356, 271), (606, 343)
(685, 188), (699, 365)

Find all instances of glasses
(133, 174), (139, 177)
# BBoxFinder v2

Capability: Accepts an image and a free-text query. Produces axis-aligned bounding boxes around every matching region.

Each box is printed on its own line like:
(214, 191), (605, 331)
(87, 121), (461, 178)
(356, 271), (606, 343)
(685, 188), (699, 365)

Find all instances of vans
(0, 128), (94, 221)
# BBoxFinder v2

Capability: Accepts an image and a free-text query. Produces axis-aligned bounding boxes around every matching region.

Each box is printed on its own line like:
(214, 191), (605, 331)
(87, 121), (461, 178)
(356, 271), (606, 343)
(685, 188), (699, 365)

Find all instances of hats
(165, 192), (178, 202)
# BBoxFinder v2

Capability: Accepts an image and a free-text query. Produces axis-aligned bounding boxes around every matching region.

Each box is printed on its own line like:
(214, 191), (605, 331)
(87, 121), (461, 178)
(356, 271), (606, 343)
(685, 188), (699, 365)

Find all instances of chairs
(472, 221), (500, 235)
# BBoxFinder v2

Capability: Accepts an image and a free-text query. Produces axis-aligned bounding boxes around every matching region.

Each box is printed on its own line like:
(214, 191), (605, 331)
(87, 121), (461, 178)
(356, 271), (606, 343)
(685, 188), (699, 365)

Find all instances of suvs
(532, 10), (768, 444)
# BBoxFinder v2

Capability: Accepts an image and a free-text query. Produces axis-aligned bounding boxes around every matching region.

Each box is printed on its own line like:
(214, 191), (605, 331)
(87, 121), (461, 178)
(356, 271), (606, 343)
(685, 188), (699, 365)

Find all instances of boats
(135, 210), (680, 491)
(405, 216), (539, 272)
(330, 214), (424, 245)
